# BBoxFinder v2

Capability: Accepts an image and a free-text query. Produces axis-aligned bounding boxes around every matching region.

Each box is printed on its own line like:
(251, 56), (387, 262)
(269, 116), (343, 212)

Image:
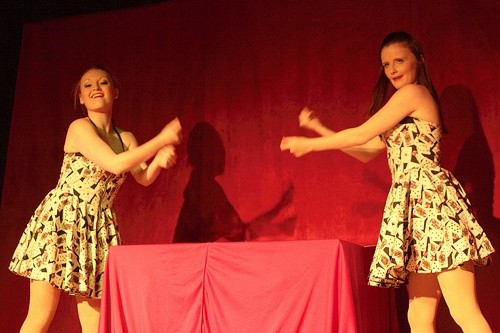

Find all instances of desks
(98, 240), (399, 333)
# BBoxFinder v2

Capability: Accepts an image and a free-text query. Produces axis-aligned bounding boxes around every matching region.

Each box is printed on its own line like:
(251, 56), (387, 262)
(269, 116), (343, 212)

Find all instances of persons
(280, 31), (495, 333)
(8, 64), (182, 333)
